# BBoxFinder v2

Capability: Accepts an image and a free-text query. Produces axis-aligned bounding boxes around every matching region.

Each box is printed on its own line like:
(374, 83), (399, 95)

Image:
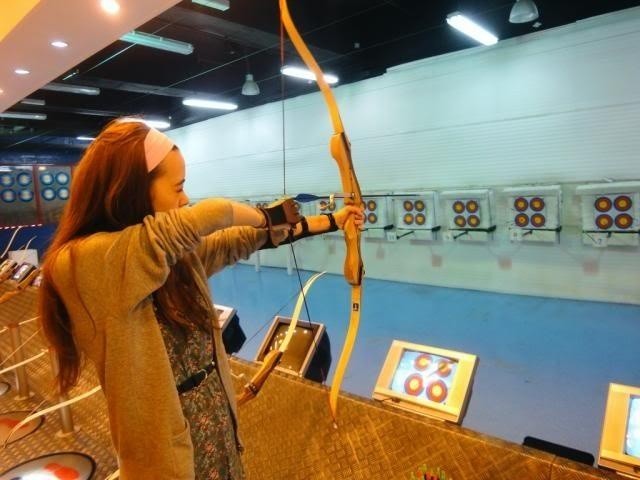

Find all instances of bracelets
(326, 213), (338, 232)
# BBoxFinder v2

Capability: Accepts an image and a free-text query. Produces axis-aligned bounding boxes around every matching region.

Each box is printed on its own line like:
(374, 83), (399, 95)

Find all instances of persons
(36, 120), (365, 480)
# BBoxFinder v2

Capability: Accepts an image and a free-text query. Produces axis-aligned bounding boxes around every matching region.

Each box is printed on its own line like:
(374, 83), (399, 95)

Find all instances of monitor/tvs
(597, 382), (640, 480)
(0, 259), (39, 288)
(253, 315), (332, 384)
(213, 304), (248, 355)
(371, 339), (479, 426)
